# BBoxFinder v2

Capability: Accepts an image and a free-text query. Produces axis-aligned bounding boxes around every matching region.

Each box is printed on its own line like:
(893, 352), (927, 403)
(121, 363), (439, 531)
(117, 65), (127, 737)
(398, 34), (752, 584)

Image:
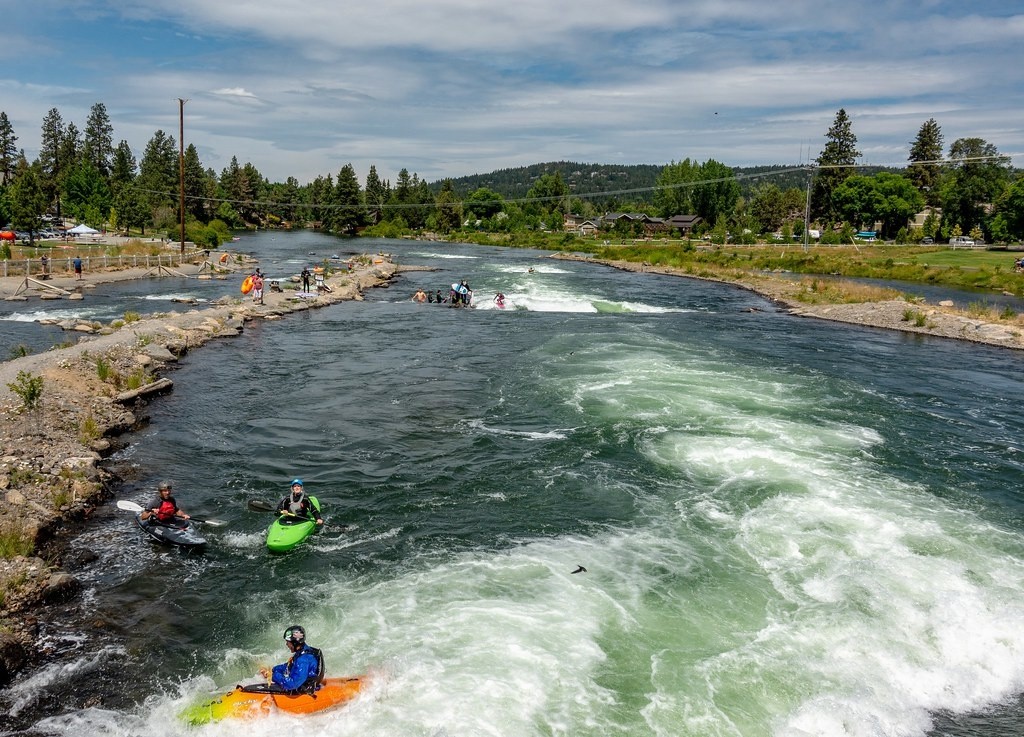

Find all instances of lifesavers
(374, 260), (383, 264)
(220, 254), (228, 261)
(241, 276), (253, 294)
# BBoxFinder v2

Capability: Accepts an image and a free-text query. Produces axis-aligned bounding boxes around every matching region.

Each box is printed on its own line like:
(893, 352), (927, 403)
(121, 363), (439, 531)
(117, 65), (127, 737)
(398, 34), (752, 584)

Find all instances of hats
(292, 479), (303, 487)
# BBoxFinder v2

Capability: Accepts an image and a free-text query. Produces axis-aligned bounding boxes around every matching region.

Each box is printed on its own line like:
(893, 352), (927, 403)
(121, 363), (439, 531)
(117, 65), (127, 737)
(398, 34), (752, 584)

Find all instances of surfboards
(452, 283), (467, 295)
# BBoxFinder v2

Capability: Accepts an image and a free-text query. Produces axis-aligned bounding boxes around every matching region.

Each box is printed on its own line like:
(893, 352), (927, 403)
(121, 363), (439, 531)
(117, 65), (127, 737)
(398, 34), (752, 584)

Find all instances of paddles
(248, 499), (349, 529)
(266, 664), (272, 689)
(117, 500), (227, 527)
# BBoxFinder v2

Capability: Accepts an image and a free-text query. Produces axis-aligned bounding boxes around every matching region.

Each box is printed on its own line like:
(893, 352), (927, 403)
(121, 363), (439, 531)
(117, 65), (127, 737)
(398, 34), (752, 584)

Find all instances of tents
(66, 223), (98, 233)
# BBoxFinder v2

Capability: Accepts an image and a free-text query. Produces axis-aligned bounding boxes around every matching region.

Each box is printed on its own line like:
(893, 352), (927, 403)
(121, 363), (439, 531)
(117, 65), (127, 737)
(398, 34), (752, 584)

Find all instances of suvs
(949, 235), (983, 245)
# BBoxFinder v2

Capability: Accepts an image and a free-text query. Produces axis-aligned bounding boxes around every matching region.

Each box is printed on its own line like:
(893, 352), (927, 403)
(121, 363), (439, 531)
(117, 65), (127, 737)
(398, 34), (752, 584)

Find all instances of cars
(12, 213), (79, 240)
(922, 236), (935, 244)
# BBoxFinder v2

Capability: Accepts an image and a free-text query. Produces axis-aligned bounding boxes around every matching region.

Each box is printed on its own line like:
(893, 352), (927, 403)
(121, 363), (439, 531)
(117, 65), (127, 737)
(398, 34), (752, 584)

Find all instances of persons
(413, 288), (427, 303)
(141, 481), (191, 527)
(260, 625), (325, 694)
(1016, 258), (1024, 271)
(73, 256), (82, 280)
(449, 278), (472, 306)
(493, 291), (505, 304)
(717, 244), (720, 250)
(273, 479), (323, 524)
(42, 252), (48, 274)
(301, 267), (311, 293)
(428, 291), (434, 303)
(252, 268), (264, 305)
(437, 289), (445, 304)
(313, 264), (318, 280)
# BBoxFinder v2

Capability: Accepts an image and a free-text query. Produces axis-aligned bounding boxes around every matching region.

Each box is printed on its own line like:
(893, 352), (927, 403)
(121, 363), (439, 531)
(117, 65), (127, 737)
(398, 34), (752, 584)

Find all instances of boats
(265, 495), (320, 556)
(131, 511), (209, 550)
(181, 670), (377, 727)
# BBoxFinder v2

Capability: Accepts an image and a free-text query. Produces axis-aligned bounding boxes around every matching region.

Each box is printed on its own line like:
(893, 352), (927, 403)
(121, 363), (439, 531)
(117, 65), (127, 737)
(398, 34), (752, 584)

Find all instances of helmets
(258, 273), (263, 277)
(158, 481), (172, 490)
(284, 626), (305, 646)
